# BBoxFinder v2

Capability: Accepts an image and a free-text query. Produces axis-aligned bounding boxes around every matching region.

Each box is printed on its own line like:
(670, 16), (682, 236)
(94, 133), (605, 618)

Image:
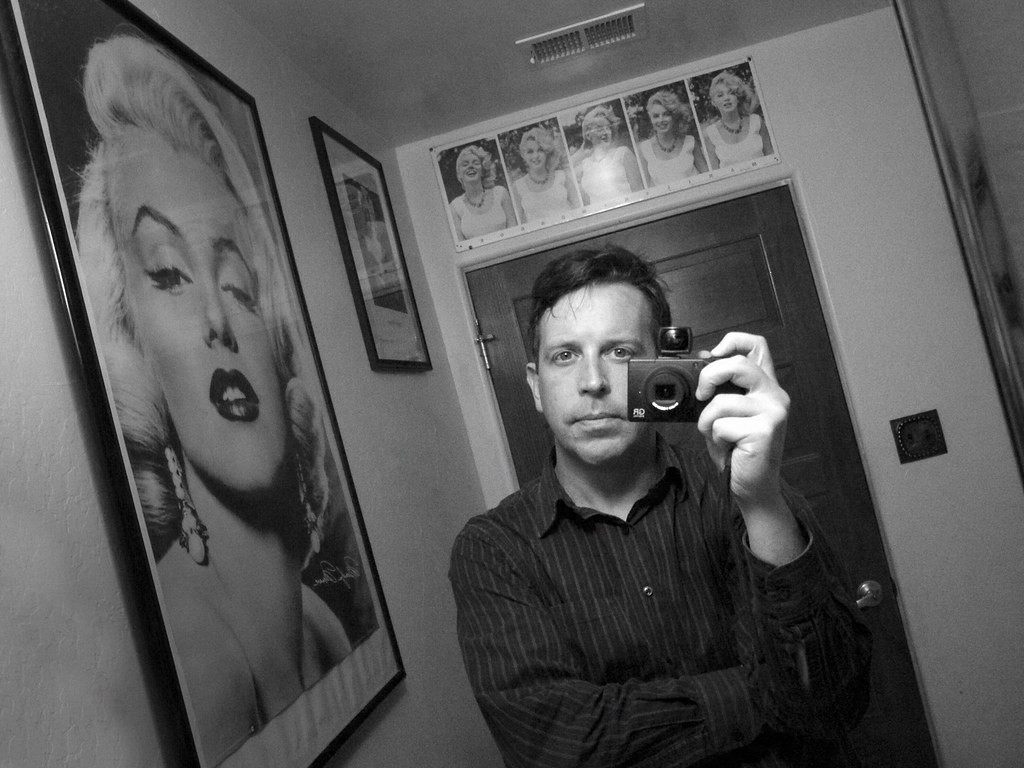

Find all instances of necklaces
(721, 114), (744, 135)
(593, 152), (606, 162)
(465, 189), (485, 209)
(529, 168), (550, 185)
(657, 134), (678, 153)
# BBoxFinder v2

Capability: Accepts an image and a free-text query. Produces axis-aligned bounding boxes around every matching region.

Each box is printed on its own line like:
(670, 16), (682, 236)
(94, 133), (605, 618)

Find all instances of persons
(568, 106), (643, 206)
(514, 127), (580, 225)
(447, 145), (516, 245)
(639, 90), (708, 189)
(702, 71), (774, 171)
(449, 245), (868, 768)
(362, 209), (384, 274)
(77, 32), (352, 765)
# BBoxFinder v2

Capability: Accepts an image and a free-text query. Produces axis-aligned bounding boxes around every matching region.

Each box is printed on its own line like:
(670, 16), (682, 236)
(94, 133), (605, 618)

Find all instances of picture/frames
(308, 115), (434, 373)
(1, 0), (407, 768)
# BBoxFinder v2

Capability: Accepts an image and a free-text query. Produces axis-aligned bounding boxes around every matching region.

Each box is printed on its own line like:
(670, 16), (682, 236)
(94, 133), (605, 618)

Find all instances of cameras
(628, 326), (746, 423)
